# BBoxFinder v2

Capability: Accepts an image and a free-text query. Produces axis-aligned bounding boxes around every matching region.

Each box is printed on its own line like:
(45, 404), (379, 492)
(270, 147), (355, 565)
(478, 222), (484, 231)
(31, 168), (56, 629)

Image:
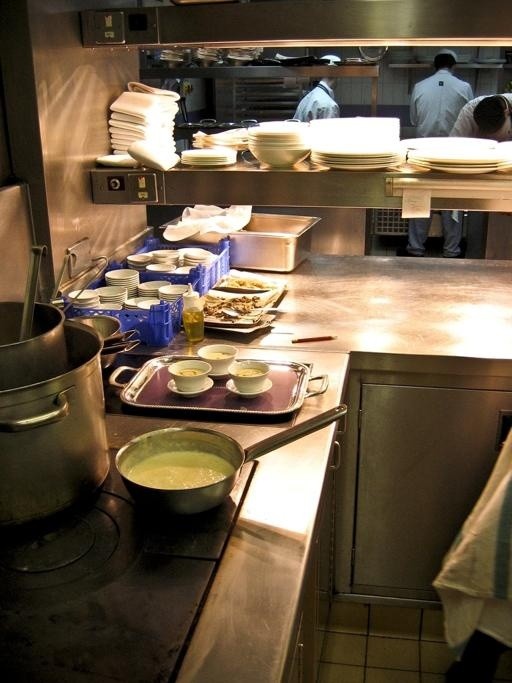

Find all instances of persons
(400, 47), (475, 258)
(445, 88), (510, 258)
(292, 51), (342, 120)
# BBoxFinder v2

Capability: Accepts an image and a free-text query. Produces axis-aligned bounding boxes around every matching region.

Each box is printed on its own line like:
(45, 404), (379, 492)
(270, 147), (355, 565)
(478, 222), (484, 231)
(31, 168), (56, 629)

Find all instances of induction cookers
(0, 446), (258, 683)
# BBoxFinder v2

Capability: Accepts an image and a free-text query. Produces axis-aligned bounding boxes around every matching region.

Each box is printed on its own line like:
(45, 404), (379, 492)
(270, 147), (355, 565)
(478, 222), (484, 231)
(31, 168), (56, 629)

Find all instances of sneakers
(445, 251), (465, 258)
(395, 248), (424, 257)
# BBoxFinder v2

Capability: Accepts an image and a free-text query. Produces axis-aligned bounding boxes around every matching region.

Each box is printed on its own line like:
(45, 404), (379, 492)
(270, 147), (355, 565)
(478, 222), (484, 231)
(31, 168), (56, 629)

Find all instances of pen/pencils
(292, 335), (336, 343)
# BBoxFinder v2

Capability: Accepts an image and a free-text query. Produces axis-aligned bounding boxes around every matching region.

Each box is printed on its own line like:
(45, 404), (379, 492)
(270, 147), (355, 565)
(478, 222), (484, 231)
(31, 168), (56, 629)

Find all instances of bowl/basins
(67, 313), (122, 370)
(247, 121), (312, 168)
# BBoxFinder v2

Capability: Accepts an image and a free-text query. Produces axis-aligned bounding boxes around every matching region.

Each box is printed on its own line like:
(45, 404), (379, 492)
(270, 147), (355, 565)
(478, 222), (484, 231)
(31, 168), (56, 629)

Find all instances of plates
(308, 136), (512, 175)
(225, 377), (274, 400)
(180, 147), (238, 167)
(167, 376), (215, 398)
(207, 361), (239, 382)
(67, 247), (213, 322)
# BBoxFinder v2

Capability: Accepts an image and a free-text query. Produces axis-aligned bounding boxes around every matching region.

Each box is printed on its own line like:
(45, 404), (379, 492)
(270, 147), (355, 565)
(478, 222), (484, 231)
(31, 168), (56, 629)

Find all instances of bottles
(182, 282), (206, 342)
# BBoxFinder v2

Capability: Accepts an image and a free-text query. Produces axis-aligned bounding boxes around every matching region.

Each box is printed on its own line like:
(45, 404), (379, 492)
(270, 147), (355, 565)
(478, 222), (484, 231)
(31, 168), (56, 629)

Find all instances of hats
(319, 55), (341, 65)
(435, 49), (457, 62)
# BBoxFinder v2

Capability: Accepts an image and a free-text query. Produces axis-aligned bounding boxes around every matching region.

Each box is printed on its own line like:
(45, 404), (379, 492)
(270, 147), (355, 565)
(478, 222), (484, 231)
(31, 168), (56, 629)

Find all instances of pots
(0, 318), (111, 532)
(113, 403), (348, 515)
(0, 301), (69, 392)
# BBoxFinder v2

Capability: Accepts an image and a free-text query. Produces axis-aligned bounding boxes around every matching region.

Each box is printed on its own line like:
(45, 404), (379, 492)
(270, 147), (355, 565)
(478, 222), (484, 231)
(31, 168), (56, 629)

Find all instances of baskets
(59, 236), (230, 348)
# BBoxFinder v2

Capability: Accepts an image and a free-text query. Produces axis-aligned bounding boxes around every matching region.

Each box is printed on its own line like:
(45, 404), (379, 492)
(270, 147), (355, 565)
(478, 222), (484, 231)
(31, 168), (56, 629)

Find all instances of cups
(197, 343), (237, 374)
(167, 359), (213, 392)
(227, 360), (270, 393)
